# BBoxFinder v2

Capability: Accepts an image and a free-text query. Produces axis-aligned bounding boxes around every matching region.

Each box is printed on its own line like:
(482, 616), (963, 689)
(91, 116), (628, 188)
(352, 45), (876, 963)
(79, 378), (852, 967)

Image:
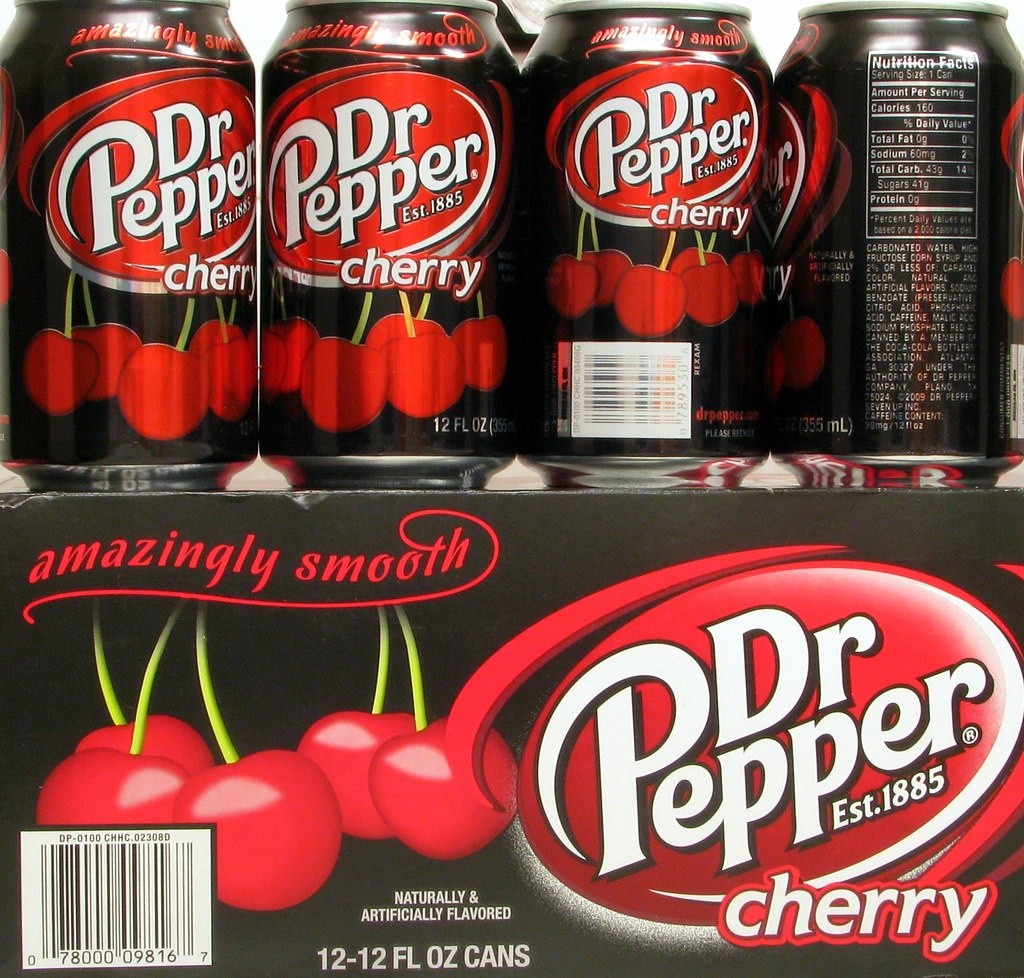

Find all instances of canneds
(776, 3), (1024, 485)
(262, 0), (521, 490)
(0, 0), (260, 491)
(521, 1), (776, 490)
